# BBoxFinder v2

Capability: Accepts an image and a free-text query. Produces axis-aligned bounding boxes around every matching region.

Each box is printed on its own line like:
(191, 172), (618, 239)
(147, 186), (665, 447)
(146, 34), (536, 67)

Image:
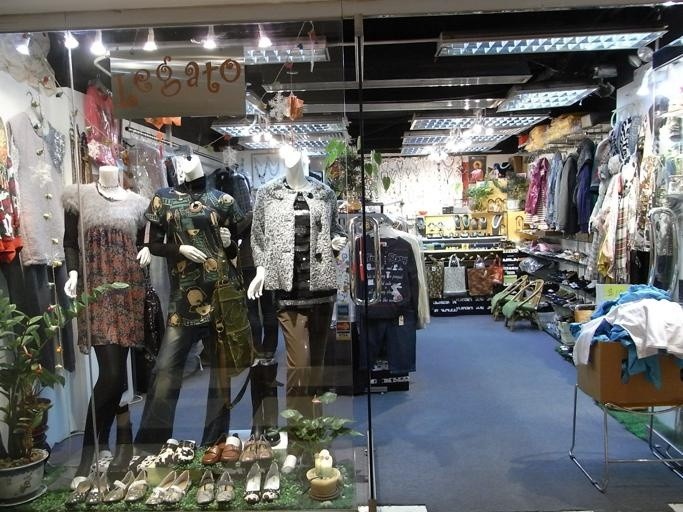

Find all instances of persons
(61, 166), (151, 491)
(130, 156), (249, 482)
(247, 154), (346, 476)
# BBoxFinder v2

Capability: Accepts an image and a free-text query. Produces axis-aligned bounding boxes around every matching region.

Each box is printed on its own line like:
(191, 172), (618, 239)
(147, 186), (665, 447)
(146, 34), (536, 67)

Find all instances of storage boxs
(574, 339), (683, 407)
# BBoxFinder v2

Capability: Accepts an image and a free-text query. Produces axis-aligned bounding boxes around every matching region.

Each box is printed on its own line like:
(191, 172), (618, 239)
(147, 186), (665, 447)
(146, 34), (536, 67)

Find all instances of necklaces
(96, 180), (118, 189)
(183, 179), (204, 214)
(453, 215), (502, 229)
(499, 163), (510, 170)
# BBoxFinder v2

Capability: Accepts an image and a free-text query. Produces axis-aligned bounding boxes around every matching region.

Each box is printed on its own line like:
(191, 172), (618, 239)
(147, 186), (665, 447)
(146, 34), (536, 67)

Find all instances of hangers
(209, 162), (231, 174)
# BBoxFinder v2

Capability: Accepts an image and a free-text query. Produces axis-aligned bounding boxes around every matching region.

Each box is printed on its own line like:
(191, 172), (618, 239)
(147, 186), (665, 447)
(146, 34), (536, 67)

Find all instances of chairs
(505, 279), (544, 330)
(491, 275), (530, 320)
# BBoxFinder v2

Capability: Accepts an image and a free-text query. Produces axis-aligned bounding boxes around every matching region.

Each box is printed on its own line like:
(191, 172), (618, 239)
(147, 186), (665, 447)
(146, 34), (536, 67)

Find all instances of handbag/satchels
(444, 266), (467, 297)
(143, 286), (164, 370)
(468, 265), (503, 296)
(213, 280), (256, 380)
(425, 264), (443, 299)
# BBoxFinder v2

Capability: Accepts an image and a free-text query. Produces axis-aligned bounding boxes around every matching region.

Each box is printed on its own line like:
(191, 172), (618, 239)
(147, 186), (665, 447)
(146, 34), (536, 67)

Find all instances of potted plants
(0, 281), (129, 508)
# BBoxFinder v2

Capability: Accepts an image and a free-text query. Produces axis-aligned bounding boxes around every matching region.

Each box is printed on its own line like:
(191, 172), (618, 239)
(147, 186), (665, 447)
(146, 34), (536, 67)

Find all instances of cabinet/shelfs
(522, 230), (600, 339)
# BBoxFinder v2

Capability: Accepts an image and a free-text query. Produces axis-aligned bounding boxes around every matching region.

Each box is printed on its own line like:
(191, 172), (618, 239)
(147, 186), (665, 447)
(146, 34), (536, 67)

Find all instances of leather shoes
(546, 293), (583, 309)
(65, 434), (282, 506)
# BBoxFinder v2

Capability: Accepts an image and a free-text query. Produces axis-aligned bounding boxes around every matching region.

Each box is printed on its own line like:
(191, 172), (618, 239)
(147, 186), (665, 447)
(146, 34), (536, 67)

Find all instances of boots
(249, 359), (280, 447)
